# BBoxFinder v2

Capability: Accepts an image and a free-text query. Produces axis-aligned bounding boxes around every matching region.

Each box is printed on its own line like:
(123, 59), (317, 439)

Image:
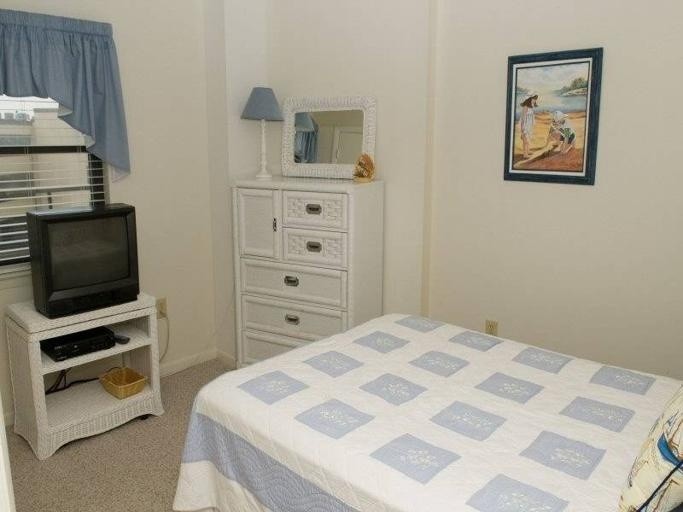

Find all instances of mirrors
(281, 94), (377, 179)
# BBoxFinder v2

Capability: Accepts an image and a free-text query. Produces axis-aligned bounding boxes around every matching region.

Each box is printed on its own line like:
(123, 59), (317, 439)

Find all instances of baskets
(101, 367), (148, 399)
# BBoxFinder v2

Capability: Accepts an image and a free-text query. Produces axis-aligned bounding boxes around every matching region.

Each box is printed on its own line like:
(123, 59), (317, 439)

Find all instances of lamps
(239, 85), (285, 179)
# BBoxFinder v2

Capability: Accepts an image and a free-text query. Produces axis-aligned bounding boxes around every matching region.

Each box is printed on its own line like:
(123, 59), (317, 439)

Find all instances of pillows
(617, 386), (683, 512)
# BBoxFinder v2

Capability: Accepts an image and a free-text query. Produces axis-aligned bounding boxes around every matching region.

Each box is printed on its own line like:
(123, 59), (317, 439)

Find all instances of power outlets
(155, 298), (167, 319)
(484, 320), (497, 337)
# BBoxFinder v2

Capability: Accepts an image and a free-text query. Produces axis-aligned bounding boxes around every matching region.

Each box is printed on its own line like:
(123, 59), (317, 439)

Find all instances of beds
(172, 313), (683, 512)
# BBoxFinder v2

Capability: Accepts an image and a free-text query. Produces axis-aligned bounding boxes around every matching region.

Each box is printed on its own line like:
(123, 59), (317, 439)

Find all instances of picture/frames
(503, 47), (603, 186)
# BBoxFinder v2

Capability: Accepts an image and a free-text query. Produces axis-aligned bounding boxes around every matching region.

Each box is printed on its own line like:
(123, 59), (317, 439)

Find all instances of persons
(519, 90), (539, 157)
(546, 110), (577, 154)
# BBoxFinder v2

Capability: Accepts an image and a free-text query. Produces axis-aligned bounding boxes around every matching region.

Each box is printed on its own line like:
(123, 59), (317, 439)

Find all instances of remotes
(115, 334), (130, 344)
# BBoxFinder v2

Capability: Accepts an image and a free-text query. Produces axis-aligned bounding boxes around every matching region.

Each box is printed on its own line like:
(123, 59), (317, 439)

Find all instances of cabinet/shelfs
(227, 176), (385, 370)
(5, 292), (166, 461)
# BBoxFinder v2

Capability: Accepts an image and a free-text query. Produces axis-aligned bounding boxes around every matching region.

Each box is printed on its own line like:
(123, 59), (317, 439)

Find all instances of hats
(552, 110), (566, 122)
(520, 94), (540, 108)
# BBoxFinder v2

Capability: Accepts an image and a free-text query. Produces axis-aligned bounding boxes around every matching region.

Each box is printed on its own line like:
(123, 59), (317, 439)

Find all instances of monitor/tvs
(26, 203), (139, 319)
